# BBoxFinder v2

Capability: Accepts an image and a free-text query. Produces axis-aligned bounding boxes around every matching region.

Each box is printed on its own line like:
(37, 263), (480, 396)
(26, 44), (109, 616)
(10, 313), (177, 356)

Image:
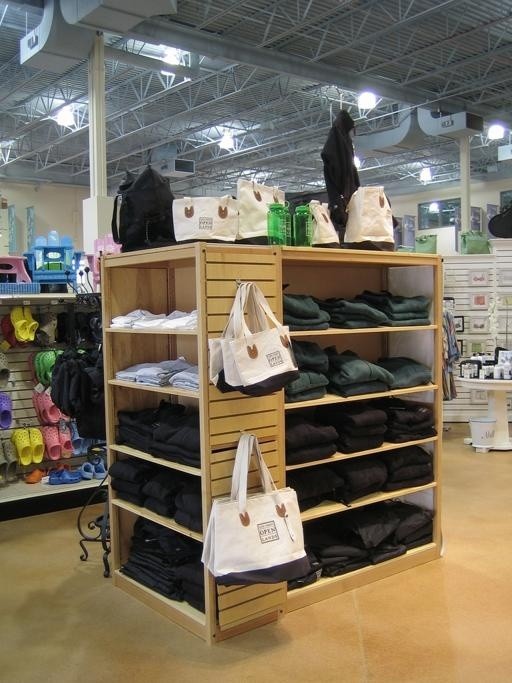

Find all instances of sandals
(11, 422), (92, 465)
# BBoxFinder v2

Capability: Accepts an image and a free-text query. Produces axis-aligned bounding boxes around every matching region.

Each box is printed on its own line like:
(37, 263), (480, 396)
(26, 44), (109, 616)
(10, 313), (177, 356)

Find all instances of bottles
(462, 351), (511, 378)
(295, 203), (313, 245)
(266, 203), (287, 243)
(285, 205), (293, 242)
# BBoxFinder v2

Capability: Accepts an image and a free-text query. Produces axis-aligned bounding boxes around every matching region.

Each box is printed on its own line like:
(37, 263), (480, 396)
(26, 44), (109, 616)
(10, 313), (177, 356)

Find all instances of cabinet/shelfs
(100, 241), (446, 643)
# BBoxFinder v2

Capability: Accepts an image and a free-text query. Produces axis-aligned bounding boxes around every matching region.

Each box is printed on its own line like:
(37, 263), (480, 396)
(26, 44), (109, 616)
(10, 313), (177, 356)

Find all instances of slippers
(0, 440), (18, 484)
(0, 392), (11, 428)
(0, 305), (58, 349)
(93, 457), (107, 480)
(32, 386), (70, 425)
(77, 461), (94, 481)
(27, 349), (65, 384)
(48, 468), (82, 486)
(0, 352), (9, 387)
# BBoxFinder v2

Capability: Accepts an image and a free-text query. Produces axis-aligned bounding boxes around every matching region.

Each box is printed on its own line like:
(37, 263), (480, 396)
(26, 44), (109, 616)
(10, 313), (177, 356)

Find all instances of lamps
(56, 100), (76, 126)
(419, 167), (432, 180)
(218, 130), (236, 149)
(158, 46), (182, 75)
(358, 91), (376, 110)
(488, 124), (504, 140)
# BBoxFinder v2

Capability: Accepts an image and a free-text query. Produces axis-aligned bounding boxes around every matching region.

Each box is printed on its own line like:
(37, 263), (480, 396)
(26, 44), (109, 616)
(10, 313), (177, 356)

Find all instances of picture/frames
(445, 269), (511, 406)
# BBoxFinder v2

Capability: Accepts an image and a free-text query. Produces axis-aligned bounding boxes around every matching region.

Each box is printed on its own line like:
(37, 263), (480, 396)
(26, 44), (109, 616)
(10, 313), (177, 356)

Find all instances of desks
(455, 374), (511, 453)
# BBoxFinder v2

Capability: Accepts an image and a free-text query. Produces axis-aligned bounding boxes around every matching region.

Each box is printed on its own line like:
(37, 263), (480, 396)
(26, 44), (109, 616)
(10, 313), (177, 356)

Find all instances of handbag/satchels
(397, 233), (437, 253)
(306, 199), (341, 249)
(343, 185), (395, 253)
(235, 177), (287, 246)
(200, 432), (313, 589)
(206, 282), (301, 398)
(459, 229), (492, 255)
(171, 194), (240, 245)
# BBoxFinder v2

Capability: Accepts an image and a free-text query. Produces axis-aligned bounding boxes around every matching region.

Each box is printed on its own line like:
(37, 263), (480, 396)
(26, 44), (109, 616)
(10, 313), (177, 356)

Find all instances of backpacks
(109, 163), (177, 254)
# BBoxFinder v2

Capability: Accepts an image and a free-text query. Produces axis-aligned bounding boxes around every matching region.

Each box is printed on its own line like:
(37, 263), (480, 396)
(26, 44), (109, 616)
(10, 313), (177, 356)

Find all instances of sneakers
(24, 466), (47, 484)
